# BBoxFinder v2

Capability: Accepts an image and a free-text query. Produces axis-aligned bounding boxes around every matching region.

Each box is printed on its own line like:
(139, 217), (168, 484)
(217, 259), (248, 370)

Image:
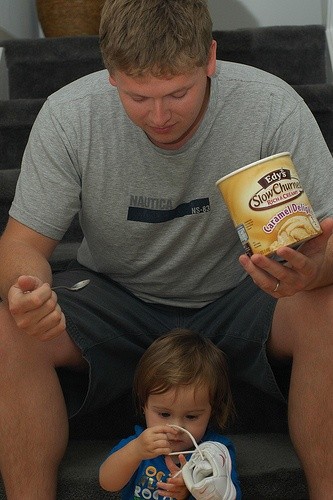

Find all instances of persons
(0, 0), (333, 500)
(96, 327), (242, 500)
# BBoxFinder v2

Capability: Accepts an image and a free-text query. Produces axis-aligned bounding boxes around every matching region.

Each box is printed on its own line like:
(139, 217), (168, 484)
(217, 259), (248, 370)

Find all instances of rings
(272, 281), (280, 293)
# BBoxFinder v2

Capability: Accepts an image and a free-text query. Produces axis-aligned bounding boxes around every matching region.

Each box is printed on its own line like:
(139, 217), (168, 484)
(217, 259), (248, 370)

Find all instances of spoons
(23, 279), (91, 293)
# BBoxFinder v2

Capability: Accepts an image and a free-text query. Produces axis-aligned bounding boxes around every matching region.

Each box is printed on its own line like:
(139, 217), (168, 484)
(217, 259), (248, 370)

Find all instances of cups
(214, 150), (324, 262)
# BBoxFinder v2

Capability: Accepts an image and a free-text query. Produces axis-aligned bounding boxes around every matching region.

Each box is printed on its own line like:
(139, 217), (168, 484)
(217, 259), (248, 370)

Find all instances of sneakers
(167, 425), (237, 500)
